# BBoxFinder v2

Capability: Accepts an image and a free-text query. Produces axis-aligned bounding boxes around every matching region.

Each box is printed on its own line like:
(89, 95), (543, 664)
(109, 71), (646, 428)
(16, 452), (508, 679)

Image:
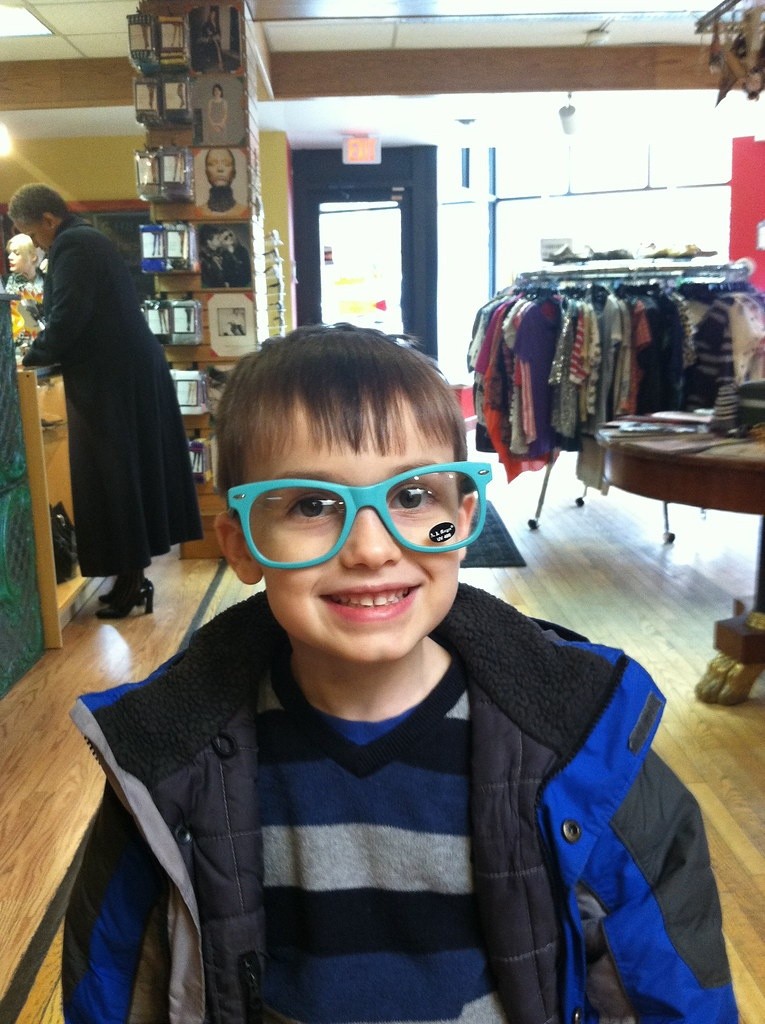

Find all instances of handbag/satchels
(49, 500), (77, 583)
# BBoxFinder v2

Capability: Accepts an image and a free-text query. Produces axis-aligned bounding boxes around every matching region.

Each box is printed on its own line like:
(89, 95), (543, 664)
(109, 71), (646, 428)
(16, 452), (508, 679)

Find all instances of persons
(62, 322), (741, 1023)
(7, 183), (204, 619)
(227, 308), (246, 335)
(203, 9), (222, 67)
(207, 83), (229, 145)
(7, 233), (47, 367)
(218, 225), (251, 287)
(195, 148), (250, 217)
(198, 224), (229, 288)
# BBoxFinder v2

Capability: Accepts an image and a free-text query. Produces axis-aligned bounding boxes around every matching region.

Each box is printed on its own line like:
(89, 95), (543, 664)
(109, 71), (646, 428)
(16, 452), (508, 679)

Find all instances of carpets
(458, 500), (526, 568)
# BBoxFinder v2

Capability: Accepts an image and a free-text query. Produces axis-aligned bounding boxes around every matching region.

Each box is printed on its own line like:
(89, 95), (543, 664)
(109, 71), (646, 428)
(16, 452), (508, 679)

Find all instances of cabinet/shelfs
(17, 362), (107, 649)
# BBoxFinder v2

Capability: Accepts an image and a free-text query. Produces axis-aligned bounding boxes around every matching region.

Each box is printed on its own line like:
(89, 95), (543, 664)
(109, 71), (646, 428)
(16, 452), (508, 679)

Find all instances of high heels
(94, 579), (153, 619)
(98, 573), (145, 607)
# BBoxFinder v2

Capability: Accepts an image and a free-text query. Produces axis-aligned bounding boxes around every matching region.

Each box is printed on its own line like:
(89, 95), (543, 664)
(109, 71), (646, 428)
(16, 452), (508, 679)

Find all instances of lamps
(558, 93), (579, 137)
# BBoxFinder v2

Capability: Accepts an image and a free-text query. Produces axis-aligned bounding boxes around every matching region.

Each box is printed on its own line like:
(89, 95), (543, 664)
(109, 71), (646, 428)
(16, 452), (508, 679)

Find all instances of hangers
(513, 265), (746, 299)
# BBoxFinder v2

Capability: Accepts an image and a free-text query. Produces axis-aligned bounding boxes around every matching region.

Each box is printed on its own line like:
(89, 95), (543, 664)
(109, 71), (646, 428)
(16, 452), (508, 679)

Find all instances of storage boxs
(464, 414), (479, 457)
(452, 384), (474, 418)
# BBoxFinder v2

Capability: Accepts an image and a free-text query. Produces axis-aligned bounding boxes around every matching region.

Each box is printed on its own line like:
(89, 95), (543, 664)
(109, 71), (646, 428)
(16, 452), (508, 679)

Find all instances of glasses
(223, 233), (232, 240)
(224, 460), (491, 568)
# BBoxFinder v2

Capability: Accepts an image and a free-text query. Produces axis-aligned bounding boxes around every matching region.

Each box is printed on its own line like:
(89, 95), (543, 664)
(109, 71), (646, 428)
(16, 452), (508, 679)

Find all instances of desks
(594, 409), (765, 709)
